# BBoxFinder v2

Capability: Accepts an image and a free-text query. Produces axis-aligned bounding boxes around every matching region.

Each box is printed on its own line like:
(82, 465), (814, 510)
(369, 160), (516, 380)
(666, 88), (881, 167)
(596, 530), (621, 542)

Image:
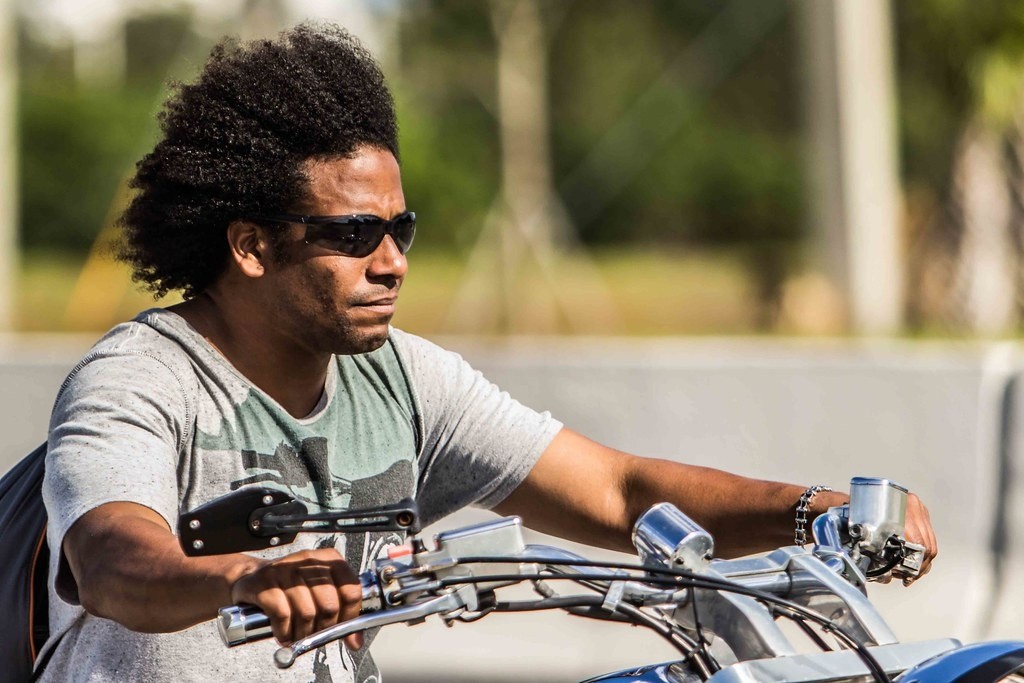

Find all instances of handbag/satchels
(0, 442), (50, 682)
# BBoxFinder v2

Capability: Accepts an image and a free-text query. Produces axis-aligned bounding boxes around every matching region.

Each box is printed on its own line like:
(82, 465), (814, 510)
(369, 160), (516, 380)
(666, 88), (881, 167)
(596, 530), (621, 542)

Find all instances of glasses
(269, 211), (416, 258)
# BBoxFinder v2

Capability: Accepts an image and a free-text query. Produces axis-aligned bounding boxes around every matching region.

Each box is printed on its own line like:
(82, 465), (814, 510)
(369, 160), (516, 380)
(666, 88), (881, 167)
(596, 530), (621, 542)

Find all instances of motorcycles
(175, 476), (1023, 683)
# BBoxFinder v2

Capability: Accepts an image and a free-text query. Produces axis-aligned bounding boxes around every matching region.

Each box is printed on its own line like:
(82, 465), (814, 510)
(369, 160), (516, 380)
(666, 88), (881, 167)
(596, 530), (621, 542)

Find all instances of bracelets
(795, 485), (830, 547)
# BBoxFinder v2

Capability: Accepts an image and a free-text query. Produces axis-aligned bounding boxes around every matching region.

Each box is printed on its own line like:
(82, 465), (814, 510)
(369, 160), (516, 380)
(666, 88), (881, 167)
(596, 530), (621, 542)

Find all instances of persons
(35, 27), (937, 683)
